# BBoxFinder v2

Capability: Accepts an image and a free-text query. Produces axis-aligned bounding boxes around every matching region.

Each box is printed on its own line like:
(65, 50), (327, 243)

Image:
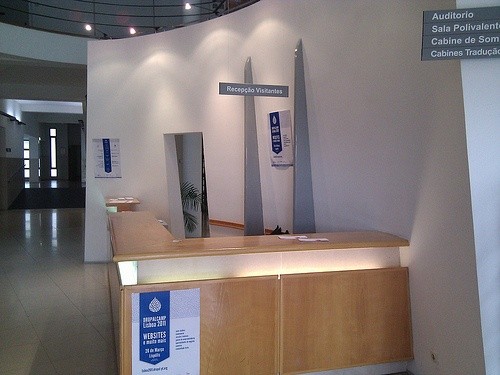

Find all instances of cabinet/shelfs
(107, 211), (414, 375)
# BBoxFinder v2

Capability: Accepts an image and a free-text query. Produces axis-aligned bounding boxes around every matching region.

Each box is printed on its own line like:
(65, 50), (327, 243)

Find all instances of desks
(105, 197), (141, 212)
